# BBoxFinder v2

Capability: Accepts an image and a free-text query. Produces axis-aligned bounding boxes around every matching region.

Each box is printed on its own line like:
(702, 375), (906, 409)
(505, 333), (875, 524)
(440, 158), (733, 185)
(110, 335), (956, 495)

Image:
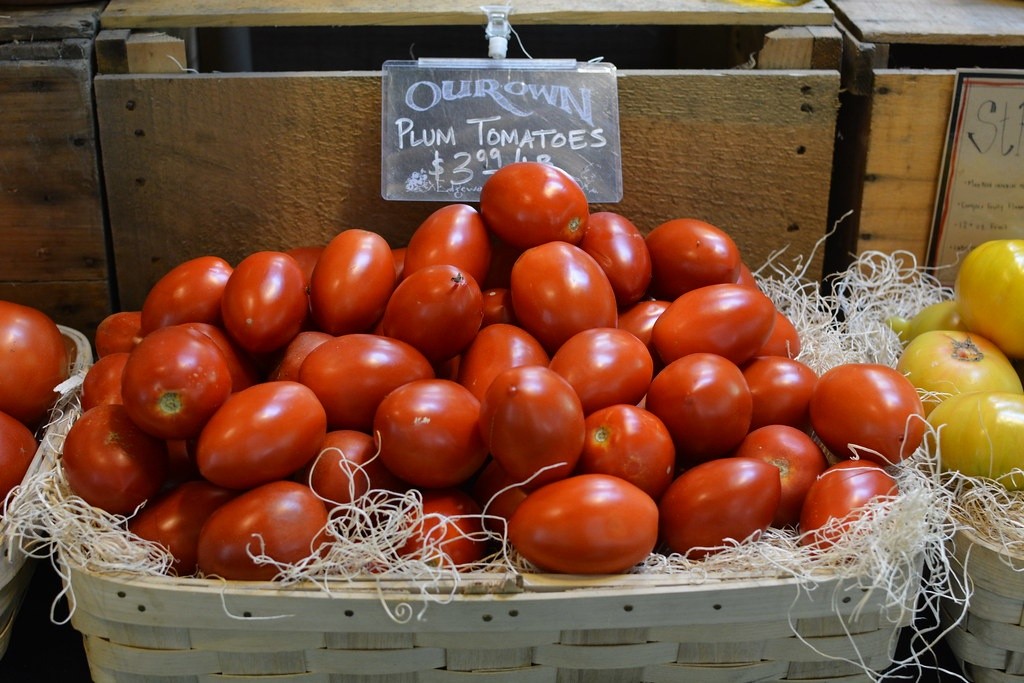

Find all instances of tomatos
(63, 165), (926, 580)
(886, 238), (1024, 492)
(0, 300), (69, 518)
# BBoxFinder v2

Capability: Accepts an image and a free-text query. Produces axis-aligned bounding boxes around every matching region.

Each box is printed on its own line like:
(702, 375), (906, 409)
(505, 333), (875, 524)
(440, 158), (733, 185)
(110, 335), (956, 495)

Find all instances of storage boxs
(0, 0), (1024, 683)
